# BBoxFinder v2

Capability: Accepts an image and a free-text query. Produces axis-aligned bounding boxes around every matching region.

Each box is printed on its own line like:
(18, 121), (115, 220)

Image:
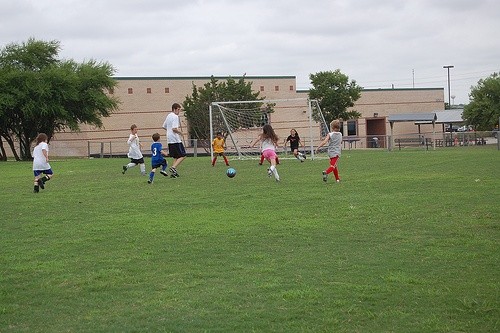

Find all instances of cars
(445, 124), (459, 132)
(456, 124), (477, 132)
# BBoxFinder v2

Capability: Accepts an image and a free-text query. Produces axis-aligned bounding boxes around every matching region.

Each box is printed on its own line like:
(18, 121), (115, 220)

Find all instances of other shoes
(38, 178), (44, 189)
(276, 180), (280, 182)
(301, 160), (304, 162)
(303, 154), (306, 159)
(169, 167), (180, 177)
(323, 171), (328, 182)
(267, 169), (273, 178)
(148, 180), (152, 184)
(171, 174), (177, 178)
(122, 166), (127, 174)
(160, 170), (168, 176)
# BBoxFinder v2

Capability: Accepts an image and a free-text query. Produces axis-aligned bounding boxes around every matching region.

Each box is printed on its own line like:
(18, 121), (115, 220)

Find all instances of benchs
(394, 138), (432, 150)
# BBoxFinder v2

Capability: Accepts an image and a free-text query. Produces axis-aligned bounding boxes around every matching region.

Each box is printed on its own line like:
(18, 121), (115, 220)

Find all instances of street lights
(443, 65), (454, 110)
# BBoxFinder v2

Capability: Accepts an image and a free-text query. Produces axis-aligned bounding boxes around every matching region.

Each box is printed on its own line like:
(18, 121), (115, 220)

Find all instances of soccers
(226, 168), (236, 178)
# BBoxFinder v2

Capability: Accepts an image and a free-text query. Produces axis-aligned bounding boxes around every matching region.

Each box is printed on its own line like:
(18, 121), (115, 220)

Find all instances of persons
(32, 133), (54, 194)
(148, 132), (169, 185)
(284, 129), (306, 163)
(314, 119), (343, 183)
(162, 102), (188, 178)
(251, 124), (282, 183)
(211, 131), (230, 167)
(372, 136), (380, 147)
(122, 124), (147, 177)
